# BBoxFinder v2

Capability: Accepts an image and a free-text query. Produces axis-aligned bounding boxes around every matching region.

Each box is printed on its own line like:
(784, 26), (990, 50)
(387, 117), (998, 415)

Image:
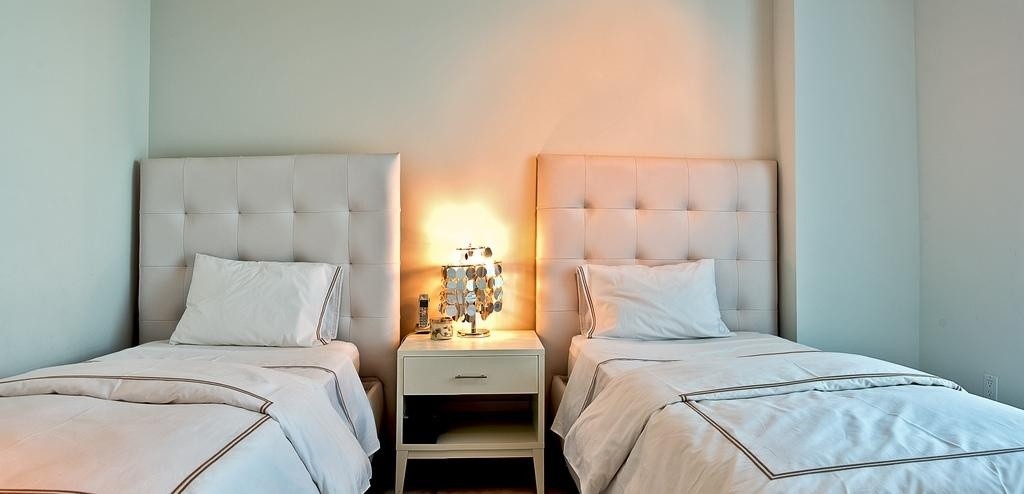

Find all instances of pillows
(170, 253), (342, 344)
(576, 259), (739, 339)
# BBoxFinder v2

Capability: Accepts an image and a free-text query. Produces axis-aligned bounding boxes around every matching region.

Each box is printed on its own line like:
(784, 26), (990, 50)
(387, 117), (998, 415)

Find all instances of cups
(429, 316), (454, 341)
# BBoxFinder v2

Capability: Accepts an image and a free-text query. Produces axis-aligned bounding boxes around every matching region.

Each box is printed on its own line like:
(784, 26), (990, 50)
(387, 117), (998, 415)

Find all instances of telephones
(414, 294), (431, 334)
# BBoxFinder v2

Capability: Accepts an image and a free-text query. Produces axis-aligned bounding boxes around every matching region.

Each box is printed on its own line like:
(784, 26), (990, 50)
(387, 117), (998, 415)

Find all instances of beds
(535, 153), (1024, 492)
(3, 151), (400, 494)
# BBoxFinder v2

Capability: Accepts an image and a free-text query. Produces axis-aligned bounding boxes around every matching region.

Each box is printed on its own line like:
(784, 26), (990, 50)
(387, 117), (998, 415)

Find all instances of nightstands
(395, 332), (548, 492)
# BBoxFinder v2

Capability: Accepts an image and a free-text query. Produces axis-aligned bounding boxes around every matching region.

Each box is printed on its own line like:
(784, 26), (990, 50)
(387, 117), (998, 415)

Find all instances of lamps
(437, 242), (505, 337)
